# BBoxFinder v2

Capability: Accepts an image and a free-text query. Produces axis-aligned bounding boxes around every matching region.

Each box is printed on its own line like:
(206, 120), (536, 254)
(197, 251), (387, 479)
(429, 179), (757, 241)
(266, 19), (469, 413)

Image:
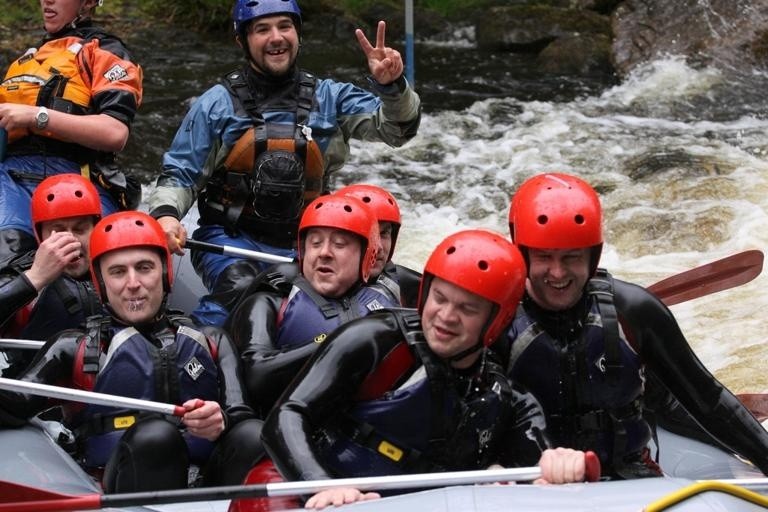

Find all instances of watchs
(36, 107), (49, 130)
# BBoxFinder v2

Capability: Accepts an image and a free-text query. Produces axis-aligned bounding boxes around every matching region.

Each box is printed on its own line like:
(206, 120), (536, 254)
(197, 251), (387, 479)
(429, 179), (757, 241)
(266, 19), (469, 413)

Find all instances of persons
(147, 1), (423, 328)
(0, 171), (768, 512)
(0, 1), (144, 271)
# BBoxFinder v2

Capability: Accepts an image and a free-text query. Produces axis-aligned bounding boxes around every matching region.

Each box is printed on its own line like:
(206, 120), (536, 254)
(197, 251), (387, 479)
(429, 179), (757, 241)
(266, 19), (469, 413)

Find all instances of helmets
(31, 173), (102, 248)
(418, 230), (528, 347)
(509, 173), (603, 277)
(233, 0), (303, 62)
(89, 211), (174, 305)
(297, 185), (401, 284)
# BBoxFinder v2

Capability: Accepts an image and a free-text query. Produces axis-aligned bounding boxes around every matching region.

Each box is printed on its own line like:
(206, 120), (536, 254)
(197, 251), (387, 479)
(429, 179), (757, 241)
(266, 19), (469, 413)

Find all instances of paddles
(645, 249), (764, 308)
(1, 450), (602, 511)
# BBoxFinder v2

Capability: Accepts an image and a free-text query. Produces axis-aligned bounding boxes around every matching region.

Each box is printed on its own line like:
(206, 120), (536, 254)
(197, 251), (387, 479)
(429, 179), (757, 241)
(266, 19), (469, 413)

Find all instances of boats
(0, 207), (768, 510)
